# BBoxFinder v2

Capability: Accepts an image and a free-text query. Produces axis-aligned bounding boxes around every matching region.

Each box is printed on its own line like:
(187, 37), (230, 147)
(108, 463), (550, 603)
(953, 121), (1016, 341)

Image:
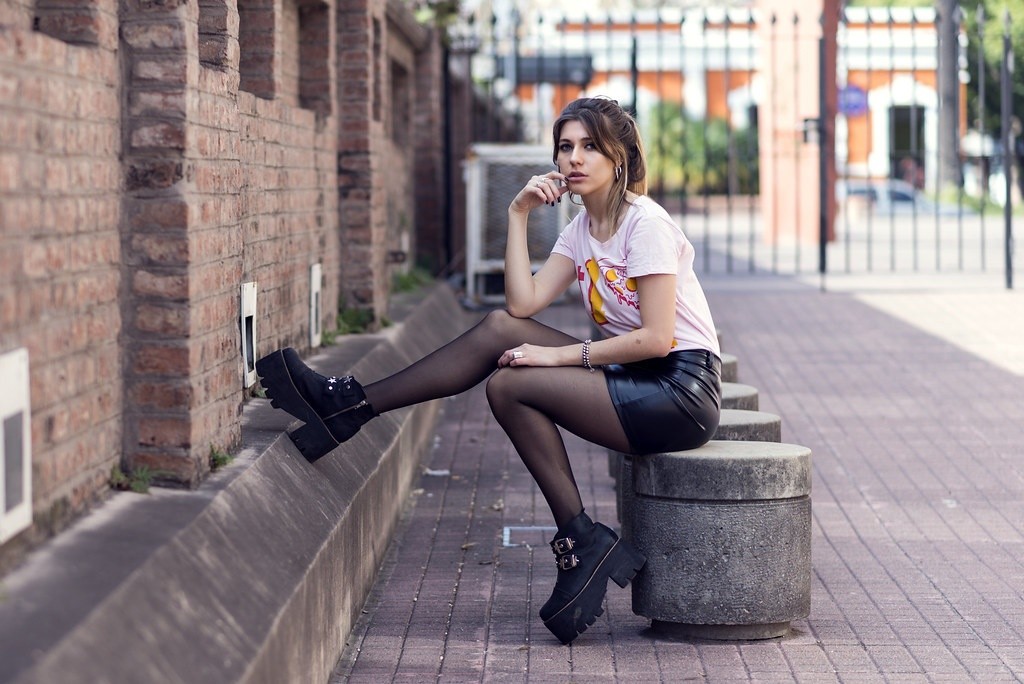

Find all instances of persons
(255, 98), (722, 644)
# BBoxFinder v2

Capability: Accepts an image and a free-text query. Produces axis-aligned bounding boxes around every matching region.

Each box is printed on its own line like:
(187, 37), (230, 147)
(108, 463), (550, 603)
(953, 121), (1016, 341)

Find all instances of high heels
(539, 508), (646, 645)
(256, 348), (380, 464)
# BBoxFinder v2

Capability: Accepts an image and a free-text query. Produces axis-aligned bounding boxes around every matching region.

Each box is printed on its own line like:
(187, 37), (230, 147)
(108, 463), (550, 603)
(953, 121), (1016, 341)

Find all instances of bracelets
(583, 339), (595, 371)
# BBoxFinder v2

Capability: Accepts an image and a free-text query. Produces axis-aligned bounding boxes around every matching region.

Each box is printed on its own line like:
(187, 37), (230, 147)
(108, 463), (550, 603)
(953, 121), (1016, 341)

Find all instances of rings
(514, 352), (523, 358)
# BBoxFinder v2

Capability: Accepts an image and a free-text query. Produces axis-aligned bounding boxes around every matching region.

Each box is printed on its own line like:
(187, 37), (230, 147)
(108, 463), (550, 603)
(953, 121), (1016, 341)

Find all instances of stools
(616, 382), (759, 525)
(619, 409), (781, 546)
(608, 353), (739, 492)
(631, 439), (811, 642)
